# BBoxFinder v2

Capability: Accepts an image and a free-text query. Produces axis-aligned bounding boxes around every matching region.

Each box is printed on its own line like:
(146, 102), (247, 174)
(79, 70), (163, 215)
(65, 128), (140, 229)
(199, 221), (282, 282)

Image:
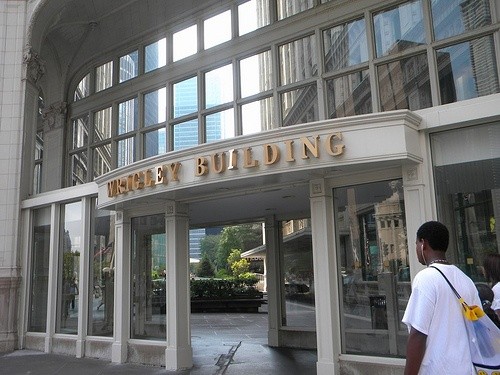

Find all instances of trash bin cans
(368, 295), (388, 330)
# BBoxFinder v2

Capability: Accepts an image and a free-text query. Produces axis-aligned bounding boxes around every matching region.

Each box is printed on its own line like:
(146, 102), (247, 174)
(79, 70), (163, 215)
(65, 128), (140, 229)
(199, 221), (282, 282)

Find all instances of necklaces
(431, 260), (447, 263)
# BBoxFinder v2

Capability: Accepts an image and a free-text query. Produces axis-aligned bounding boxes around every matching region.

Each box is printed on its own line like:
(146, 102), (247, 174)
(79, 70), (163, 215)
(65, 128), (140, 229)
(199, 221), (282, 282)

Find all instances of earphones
(421, 244), (424, 252)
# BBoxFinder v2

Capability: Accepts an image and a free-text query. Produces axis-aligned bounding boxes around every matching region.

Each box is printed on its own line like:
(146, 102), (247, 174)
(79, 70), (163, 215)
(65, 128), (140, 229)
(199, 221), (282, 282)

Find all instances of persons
(190, 273), (196, 279)
(97, 276), (105, 310)
(401, 221), (500, 375)
(67, 276), (79, 310)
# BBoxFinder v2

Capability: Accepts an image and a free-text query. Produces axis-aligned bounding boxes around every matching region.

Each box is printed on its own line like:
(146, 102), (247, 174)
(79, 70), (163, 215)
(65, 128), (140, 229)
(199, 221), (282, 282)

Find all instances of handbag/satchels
(462, 305), (500, 375)
